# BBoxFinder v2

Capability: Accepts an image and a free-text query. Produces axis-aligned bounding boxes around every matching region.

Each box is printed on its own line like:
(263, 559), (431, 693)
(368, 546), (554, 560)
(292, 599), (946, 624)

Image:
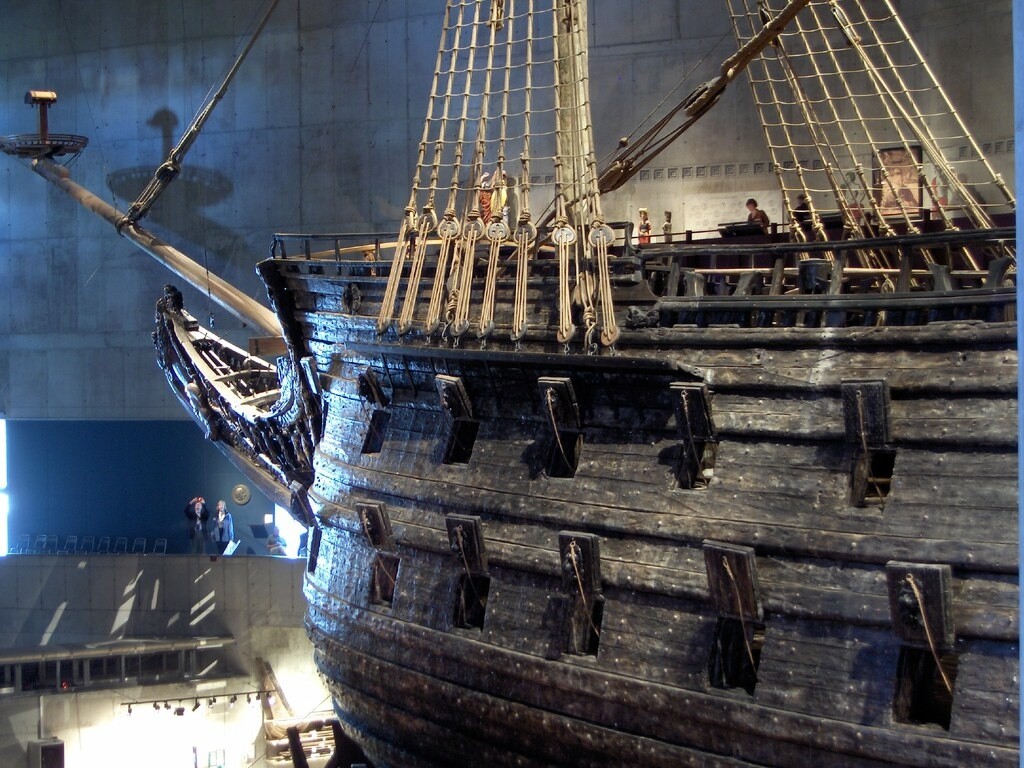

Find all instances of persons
(267, 526), (286, 556)
(476, 171), (864, 246)
(297, 532), (309, 557)
(210, 500), (234, 554)
(183, 497), (210, 555)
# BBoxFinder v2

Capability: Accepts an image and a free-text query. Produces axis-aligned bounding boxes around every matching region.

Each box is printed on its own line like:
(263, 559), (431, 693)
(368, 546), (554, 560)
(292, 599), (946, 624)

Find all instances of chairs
(154, 539), (167, 554)
(9, 534), (127, 555)
(132, 537), (146, 553)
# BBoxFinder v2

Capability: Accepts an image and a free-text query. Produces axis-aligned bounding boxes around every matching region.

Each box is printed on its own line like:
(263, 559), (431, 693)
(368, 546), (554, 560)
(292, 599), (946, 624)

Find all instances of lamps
(127, 691), (275, 715)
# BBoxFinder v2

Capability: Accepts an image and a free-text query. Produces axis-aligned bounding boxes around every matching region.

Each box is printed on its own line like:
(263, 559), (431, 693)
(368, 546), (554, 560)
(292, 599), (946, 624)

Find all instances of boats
(0, 0), (1024, 768)
